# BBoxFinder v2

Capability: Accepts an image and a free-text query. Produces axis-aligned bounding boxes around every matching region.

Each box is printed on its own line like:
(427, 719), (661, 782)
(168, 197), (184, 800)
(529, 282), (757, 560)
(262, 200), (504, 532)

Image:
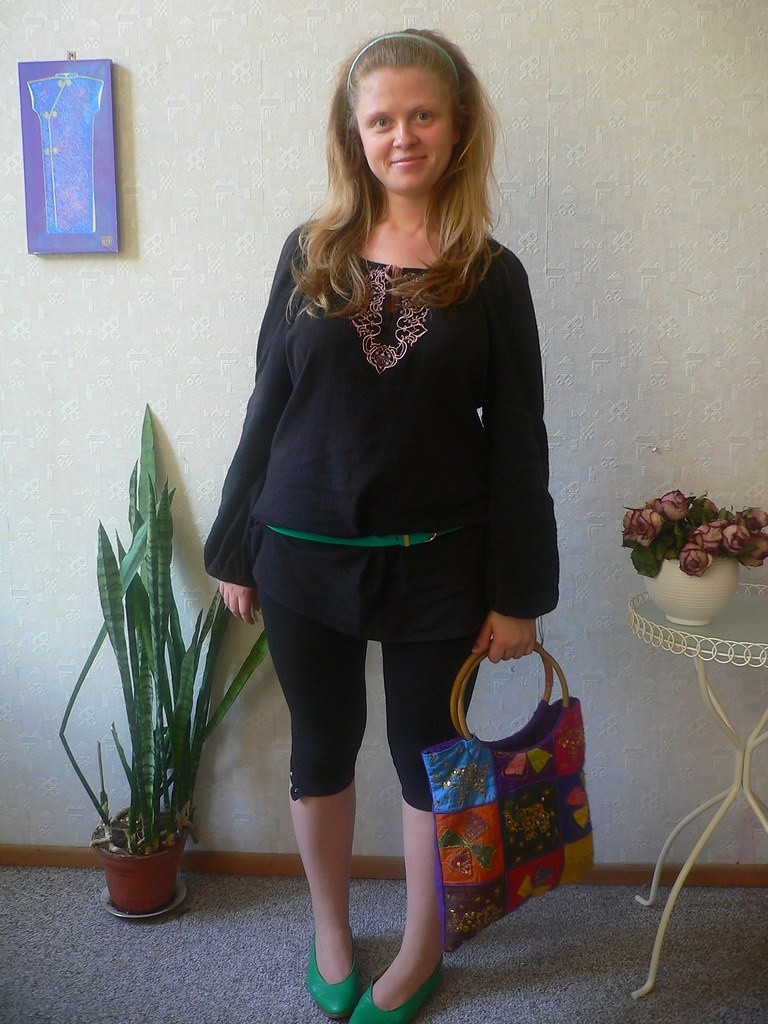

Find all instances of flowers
(621, 489), (768, 577)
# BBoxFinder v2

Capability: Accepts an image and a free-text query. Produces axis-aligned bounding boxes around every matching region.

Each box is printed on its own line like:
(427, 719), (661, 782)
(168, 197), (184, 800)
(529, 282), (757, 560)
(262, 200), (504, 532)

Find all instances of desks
(627, 582), (768, 1000)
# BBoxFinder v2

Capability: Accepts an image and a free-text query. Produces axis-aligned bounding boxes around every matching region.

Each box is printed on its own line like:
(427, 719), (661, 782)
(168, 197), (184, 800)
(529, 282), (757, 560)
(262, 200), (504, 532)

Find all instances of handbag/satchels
(419, 639), (595, 951)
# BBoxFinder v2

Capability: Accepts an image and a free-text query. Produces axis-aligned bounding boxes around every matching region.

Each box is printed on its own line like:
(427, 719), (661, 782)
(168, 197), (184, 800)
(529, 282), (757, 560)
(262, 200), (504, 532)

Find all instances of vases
(644, 556), (739, 627)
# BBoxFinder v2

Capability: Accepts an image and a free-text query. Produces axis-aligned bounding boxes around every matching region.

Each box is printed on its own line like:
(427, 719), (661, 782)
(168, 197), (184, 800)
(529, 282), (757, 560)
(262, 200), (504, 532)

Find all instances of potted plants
(59, 401), (273, 918)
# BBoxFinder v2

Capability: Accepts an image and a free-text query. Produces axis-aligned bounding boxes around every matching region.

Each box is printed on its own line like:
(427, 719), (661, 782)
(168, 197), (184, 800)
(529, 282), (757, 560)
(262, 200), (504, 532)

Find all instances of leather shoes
(306, 924), (359, 1019)
(348, 948), (443, 1024)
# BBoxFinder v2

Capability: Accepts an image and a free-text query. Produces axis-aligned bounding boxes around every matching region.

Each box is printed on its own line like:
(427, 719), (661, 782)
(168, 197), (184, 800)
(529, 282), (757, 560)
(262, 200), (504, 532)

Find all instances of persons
(201, 30), (559, 1024)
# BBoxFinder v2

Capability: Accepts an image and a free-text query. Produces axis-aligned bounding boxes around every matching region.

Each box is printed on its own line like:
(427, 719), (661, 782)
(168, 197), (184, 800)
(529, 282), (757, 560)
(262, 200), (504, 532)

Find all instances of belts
(262, 521), (462, 547)
